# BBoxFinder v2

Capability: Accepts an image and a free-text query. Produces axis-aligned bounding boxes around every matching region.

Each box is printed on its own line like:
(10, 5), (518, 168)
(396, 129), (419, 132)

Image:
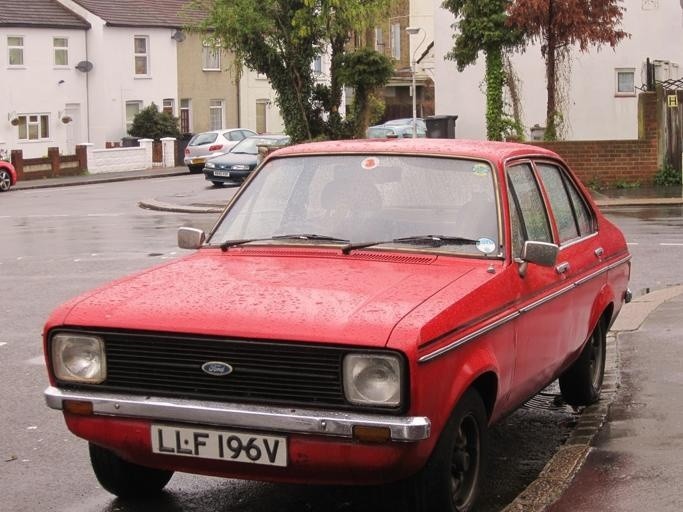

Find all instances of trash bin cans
(422, 115), (457, 138)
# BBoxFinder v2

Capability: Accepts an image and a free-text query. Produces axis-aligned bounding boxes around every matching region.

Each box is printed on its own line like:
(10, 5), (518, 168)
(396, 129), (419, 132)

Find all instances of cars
(184, 128), (292, 186)
(42, 138), (632, 509)
(365, 117), (426, 138)
(0, 162), (17, 191)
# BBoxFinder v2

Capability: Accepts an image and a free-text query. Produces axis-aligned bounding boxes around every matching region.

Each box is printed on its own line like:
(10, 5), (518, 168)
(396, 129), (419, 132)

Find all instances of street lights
(406, 25), (427, 138)
